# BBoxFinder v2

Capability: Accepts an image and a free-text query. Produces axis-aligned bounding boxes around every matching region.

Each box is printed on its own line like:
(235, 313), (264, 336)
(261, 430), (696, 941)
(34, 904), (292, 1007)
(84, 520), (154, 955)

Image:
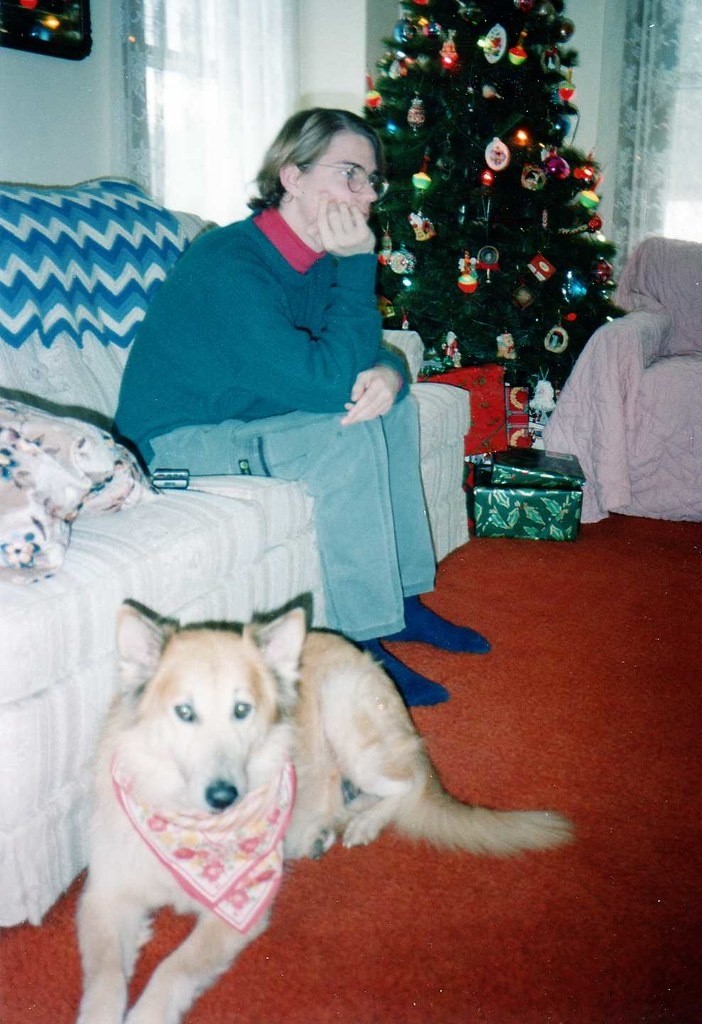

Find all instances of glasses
(297, 163), (382, 194)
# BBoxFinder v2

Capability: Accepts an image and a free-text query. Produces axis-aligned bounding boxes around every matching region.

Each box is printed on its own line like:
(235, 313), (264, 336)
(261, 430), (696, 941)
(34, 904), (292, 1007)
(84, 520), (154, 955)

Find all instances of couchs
(540, 234), (702, 520)
(1, 177), (472, 933)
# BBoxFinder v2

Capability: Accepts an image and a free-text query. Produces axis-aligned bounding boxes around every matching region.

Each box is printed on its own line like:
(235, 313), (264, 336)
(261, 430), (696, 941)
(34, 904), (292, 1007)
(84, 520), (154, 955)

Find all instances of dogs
(73, 599), (575, 1024)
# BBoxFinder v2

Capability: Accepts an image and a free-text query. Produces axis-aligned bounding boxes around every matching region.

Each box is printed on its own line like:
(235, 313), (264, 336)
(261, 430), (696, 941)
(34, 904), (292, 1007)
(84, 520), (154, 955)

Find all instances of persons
(112, 107), (493, 707)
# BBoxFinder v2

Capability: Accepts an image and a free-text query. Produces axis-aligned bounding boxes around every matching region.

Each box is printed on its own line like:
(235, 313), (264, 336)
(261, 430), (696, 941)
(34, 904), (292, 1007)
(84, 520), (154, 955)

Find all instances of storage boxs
(424, 364), (586, 544)
